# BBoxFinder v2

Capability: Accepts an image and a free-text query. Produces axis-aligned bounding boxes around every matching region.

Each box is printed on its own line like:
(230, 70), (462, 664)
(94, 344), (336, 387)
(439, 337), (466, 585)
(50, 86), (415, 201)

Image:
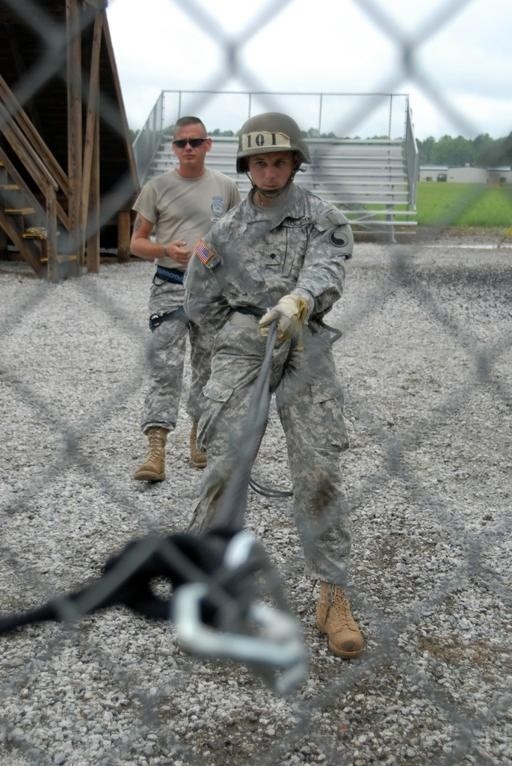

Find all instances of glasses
(173, 139), (206, 147)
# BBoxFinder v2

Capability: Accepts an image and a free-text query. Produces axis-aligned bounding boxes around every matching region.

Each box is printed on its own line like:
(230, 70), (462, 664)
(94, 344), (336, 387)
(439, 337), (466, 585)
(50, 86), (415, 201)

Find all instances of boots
(317, 583), (365, 658)
(191, 422), (207, 468)
(135, 428), (167, 482)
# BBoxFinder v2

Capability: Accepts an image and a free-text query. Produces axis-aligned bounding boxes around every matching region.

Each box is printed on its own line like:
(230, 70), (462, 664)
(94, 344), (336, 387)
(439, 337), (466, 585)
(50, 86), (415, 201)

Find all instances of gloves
(259, 294), (307, 342)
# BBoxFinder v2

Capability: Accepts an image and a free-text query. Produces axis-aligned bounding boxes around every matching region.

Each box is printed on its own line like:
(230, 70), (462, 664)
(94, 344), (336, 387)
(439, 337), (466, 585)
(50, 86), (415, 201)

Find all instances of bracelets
(163, 244), (169, 257)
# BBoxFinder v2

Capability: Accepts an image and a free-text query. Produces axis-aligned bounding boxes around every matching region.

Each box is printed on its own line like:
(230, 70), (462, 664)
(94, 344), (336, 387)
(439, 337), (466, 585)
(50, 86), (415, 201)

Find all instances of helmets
(236, 112), (311, 173)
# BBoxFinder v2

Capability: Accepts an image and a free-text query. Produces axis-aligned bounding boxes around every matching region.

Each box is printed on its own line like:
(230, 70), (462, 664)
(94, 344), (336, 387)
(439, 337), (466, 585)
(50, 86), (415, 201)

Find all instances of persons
(128, 114), (242, 483)
(181, 108), (366, 659)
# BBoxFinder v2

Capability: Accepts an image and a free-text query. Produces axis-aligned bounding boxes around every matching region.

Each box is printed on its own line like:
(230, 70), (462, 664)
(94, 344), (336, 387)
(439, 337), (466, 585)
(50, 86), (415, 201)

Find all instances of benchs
(136, 91), (418, 244)
